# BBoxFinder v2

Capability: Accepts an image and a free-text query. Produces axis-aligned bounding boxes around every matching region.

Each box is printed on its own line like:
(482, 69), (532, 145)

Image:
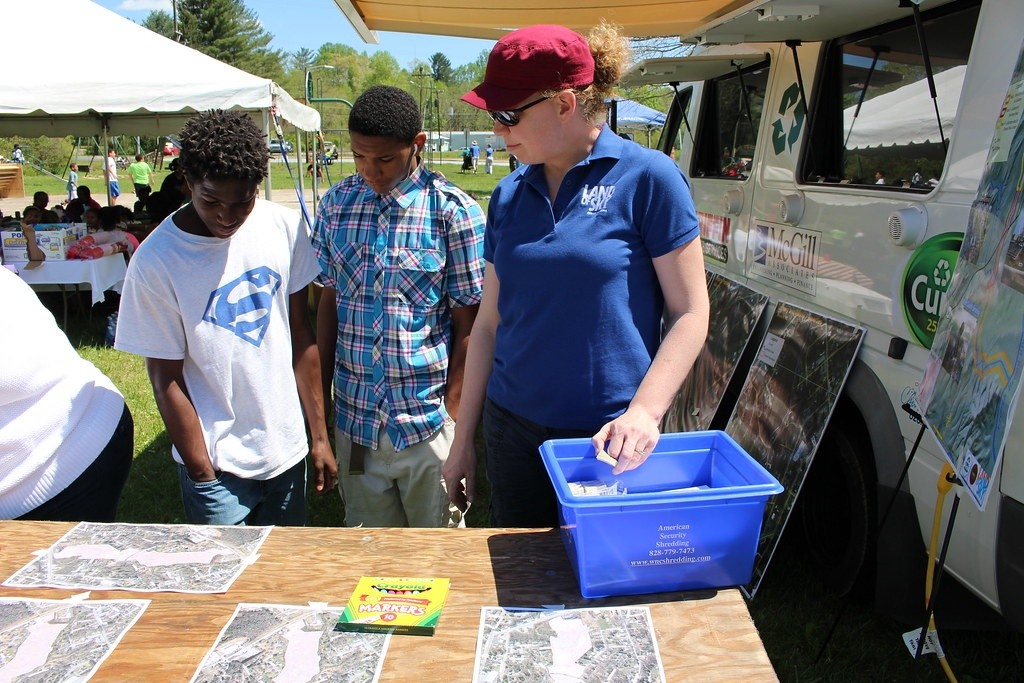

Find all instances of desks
(4, 252), (127, 334)
(0, 518), (786, 683)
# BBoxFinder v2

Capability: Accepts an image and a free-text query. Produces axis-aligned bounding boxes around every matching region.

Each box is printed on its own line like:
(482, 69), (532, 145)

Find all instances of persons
(160, 158), (187, 199)
(11, 144), (23, 160)
(0, 264), (135, 522)
(103, 149), (120, 206)
(114, 108), (337, 526)
(912, 168), (939, 187)
(307, 155), (324, 201)
(128, 154), (155, 200)
(470, 140), (480, 174)
(875, 171), (885, 184)
(309, 85), (487, 528)
(326, 142), (338, 160)
(66, 163), (78, 201)
(23, 186), (175, 240)
(444, 23), (710, 528)
(724, 147), (730, 157)
(486, 143), (494, 175)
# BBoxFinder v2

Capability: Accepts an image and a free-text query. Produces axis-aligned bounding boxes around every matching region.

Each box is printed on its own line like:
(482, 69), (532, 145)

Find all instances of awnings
(332, 0), (736, 46)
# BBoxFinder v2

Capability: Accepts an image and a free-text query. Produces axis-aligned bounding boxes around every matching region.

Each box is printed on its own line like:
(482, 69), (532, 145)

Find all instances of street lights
(304, 65), (335, 163)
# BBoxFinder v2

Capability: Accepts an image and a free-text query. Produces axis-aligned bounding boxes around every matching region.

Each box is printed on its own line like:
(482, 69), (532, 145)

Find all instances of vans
(613, 0), (1024, 636)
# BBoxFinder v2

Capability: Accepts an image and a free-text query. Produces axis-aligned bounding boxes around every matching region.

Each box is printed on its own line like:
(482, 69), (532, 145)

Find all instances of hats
(472, 140), (478, 145)
(460, 24), (595, 111)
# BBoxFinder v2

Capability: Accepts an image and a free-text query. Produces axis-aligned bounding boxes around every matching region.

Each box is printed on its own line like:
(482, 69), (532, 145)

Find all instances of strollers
(460, 153), (475, 174)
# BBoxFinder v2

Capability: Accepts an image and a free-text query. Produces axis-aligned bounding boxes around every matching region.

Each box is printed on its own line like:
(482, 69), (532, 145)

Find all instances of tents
(604, 94), (667, 149)
(0, 0), (321, 200)
(844, 64), (968, 150)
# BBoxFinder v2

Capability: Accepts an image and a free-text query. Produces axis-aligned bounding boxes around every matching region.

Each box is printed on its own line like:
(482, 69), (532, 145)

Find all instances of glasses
(487, 95), (552, 127)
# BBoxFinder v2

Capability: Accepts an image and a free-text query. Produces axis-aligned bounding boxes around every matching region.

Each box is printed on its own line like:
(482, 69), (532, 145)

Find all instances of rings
(634, 448), (645, 455)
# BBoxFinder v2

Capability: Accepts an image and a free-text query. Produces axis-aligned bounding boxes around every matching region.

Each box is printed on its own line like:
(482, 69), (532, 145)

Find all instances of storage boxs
(537, 428), (784, 600)
(1, 223), (87, 262)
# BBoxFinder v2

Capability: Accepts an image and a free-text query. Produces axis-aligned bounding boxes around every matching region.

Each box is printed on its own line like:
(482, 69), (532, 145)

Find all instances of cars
(454, 146), (470, 151)
(269, 138), (294, 154)
(316, 141), (339, 160)
